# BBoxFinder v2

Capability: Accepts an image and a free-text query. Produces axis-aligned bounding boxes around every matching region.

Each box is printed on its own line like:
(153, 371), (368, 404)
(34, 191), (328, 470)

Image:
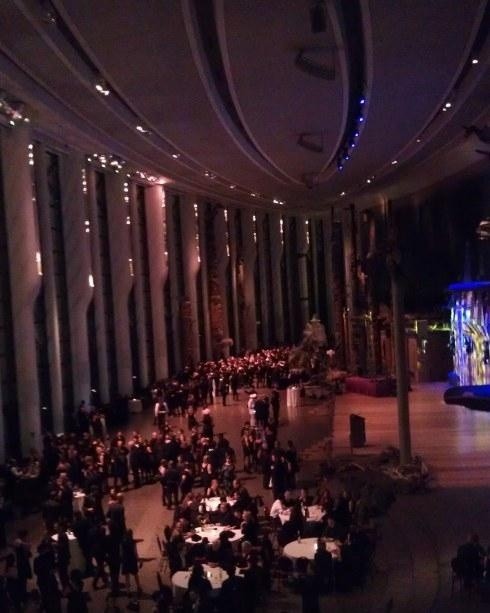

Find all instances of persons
(0, 343), (374, 610)
(454, 529), (488, 591)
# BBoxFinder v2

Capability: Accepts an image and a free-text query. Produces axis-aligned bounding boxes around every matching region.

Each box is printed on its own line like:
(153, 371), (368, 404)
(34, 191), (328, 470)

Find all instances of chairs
(154, 483), (353, 609)
